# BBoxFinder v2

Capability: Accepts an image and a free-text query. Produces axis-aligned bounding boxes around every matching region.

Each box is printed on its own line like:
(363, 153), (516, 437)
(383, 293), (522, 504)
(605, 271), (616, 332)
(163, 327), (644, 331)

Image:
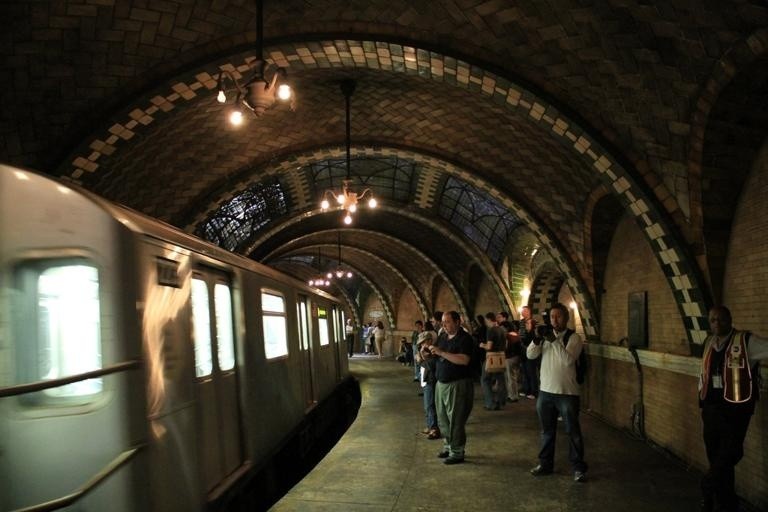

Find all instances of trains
(1, 165), (351, 510)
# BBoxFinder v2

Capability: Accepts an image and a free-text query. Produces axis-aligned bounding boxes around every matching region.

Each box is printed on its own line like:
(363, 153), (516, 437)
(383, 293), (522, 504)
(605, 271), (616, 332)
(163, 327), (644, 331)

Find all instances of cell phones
(424, 347), (431, 354)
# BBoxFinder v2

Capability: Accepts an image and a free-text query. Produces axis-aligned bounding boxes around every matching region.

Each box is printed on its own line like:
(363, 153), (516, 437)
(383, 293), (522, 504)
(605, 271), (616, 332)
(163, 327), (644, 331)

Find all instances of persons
(360, 320), (387, 359)
(526, 302), (589, 481)
(697, 306), (768, 512)
(345, 318), (355, 358)
(395, 306), (563, 464)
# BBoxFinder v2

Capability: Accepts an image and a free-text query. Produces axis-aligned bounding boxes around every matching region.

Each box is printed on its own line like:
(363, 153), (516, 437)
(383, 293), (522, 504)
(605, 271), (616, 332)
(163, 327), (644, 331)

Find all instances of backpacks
(563, 328), (587, 385)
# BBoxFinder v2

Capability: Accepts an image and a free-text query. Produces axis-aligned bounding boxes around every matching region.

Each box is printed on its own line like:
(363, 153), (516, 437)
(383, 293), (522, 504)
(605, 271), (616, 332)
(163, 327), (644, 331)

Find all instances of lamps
(320, 77), (379, 226)
(325, 231), (353, 280)
(216, 0), (292, 127)
(307, 247), (331, 287)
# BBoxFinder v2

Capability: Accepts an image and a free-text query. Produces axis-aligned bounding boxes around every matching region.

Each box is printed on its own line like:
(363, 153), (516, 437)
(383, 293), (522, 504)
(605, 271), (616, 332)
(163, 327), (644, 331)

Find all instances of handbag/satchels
(484, 351), (508, 374)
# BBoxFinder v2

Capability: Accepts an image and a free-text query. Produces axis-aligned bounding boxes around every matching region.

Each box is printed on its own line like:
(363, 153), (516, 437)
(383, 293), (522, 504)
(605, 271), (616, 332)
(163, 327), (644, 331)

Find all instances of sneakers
(530, 464), (554, 475)
(574, 470), (589, 482)
(436, 449), (450, 457)
(395, 360), (439, 440)
(484, 392), (537, 411)
(443, 455), (464, 464)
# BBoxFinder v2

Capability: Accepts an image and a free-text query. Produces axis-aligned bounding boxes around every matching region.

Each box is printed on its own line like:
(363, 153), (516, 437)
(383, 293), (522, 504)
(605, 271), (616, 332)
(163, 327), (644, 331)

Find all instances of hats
(416, 330), (432, 344)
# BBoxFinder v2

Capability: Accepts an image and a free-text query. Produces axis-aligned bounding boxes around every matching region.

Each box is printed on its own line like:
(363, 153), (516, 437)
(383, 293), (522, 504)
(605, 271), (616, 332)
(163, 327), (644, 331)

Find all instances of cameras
(531, 320), (537, 326)
(537, 325), (554, 336)
